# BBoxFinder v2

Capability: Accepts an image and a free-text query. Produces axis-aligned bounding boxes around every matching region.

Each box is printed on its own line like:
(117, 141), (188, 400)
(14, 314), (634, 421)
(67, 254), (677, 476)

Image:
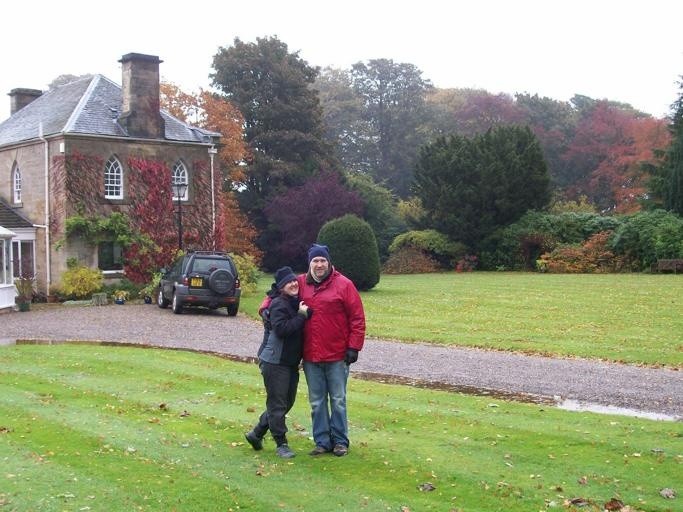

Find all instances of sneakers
(333, 443), (347, 456)
(245, 430), (263, 451)
(309, 446), (327, 455)
(276, 445), (296, 458)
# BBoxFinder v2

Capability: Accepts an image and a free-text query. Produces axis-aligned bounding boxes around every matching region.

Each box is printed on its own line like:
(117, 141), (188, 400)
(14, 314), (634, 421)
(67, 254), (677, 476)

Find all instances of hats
(308, 243), (330, 264)
(274, 267), (297, 287)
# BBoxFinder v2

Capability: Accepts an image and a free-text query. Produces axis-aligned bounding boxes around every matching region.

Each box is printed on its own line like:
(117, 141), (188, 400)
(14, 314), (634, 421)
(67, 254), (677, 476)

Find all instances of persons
(244, 266), (309, 457)
(258, 244), (365, 456)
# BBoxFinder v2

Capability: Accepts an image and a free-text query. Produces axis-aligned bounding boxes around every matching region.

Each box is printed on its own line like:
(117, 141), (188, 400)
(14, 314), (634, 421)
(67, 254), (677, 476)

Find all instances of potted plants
(13, 279), (33, 312)
(112, 289), (130, 304)
(47, 282), (64, 302)
(143, 286), (151, 303)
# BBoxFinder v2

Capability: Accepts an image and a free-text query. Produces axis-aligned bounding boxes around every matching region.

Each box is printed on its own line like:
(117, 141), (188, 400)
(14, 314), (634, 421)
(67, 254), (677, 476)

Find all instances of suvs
(158, 250), (241, 316)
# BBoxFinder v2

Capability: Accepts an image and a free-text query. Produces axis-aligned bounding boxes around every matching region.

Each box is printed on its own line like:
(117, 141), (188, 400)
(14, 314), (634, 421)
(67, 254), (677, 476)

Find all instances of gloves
(344, 349), (358, 365)
(262, 310), (270, 322)
